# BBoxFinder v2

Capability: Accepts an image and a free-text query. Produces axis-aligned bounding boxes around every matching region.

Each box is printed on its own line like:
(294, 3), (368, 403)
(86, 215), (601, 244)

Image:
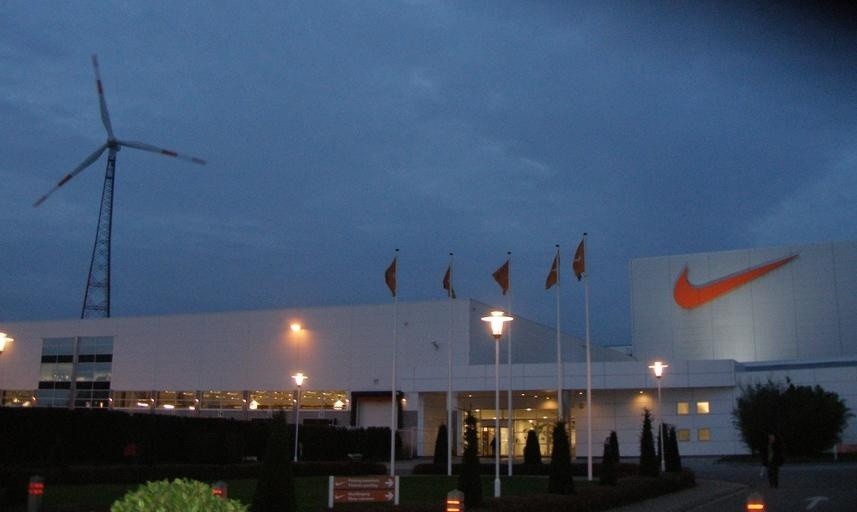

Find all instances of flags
(442, 262), (456, 301)
(491, 259), (509, 296)
(545, 249), (558, 288)
(384, 259), (396, 298)
(572, 239), (583, 279)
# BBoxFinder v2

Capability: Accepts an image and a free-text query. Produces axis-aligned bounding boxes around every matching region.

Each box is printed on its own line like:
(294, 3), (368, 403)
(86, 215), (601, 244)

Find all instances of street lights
(481, 310), (514, 499)
(291, 322), (310, 462)
(648, 361), (669, 472)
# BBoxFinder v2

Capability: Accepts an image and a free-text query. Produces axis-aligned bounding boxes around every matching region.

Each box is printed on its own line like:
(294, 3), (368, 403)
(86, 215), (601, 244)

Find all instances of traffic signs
(334, 476), (395, 502)
